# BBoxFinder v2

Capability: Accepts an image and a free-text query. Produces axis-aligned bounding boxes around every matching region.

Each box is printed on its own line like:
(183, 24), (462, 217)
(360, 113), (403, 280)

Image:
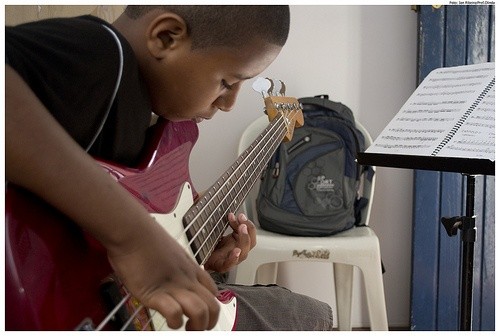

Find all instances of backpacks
(254, 94), (375, 237)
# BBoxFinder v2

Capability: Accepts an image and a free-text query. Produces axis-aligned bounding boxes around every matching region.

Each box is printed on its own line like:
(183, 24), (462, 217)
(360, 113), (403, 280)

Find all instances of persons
(7, 5), (336, 330)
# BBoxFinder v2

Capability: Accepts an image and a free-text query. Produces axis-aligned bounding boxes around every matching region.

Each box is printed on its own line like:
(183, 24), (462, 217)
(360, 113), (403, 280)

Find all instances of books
(362, 63), (495, 163)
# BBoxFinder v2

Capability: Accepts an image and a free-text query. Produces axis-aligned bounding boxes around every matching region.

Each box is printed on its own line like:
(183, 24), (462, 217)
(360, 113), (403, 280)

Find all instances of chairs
(235, 115), (390, 331)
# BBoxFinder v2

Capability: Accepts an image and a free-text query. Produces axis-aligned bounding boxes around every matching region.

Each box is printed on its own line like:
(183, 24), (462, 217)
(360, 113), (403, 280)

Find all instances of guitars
(4, 79), (305, 331)
